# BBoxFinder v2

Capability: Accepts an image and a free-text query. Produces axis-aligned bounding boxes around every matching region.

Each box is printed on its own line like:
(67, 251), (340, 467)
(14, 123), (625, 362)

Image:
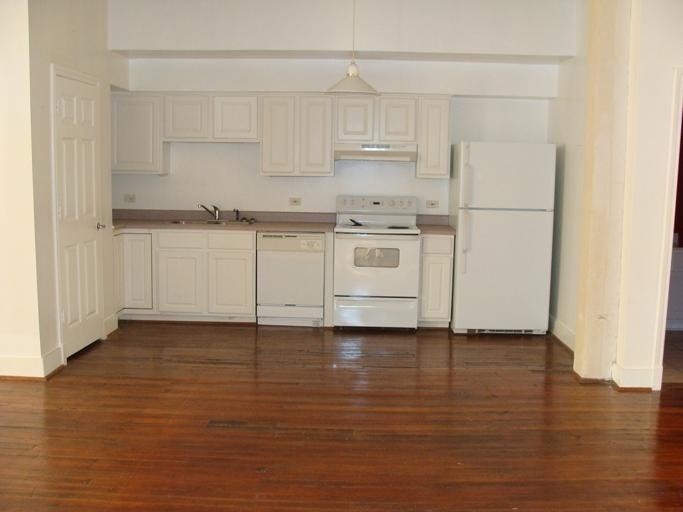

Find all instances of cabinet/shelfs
(416, 94), (453, 179)
(334, 92), (417, 145)
(163, 91), (260, 143)
(113, 228), (155, 321)
(155, 229), (257, 323)
(112, 91), (170, 175)
(417, 234), (455, 328)
(260, 91), (335, 177)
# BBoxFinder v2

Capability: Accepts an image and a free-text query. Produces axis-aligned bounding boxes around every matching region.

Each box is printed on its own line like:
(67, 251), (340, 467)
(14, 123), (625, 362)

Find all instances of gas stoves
(334, 219), (421, 234)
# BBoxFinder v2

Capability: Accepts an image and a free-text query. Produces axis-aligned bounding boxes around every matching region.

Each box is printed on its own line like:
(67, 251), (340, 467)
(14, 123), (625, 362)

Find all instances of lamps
(325, 0), (378, 93)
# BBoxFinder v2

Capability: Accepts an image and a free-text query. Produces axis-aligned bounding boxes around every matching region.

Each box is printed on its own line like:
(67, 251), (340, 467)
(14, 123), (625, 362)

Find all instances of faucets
(197, 202), (220, 219)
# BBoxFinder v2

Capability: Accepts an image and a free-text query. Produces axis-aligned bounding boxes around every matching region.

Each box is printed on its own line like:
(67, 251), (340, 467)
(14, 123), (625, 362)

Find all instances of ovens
(333, 235), (421, 328)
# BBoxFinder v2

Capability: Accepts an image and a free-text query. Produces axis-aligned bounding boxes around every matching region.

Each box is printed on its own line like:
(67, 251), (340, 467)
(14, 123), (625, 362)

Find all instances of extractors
(334, 144), (418, 163)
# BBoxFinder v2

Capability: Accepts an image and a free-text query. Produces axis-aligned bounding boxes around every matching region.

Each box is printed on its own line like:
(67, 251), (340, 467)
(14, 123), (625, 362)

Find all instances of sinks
(206, 219), (225, 225)
(172, 218), (197, 225)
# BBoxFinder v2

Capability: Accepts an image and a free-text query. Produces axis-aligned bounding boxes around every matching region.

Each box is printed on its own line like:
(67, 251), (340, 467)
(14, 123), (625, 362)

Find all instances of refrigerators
(449, 140), (558, 338)
